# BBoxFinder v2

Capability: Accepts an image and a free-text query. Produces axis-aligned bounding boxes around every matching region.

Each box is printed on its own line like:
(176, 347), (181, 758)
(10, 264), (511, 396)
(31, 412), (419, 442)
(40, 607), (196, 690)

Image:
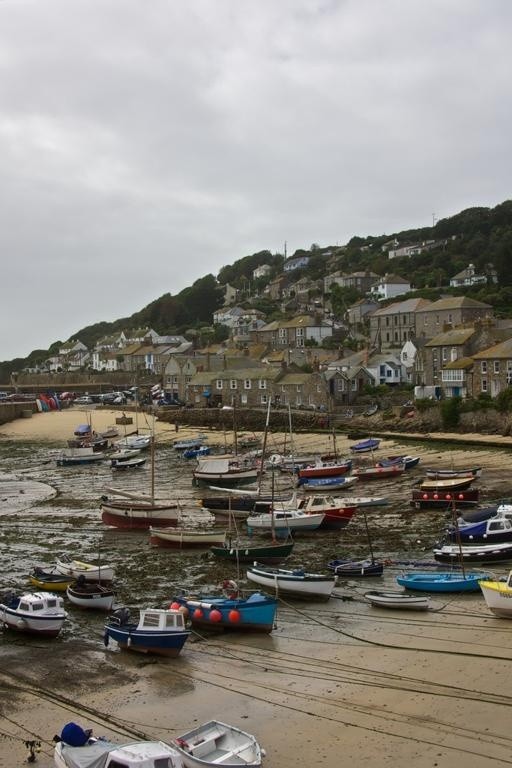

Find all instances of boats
(52, 556), (118, 583)
(29, 565), (77, 591)
(363, 586), (430, 612)
(408, 467), (485, 510)
(176, 715), (263, 767)
(246, 557), (341, 607)
(99, 602), (193, 661)
(427, 502), (512, 564)
(476, 569), (512, 617)
(51, 718), (185, 768)
(1, 587), (70, 636)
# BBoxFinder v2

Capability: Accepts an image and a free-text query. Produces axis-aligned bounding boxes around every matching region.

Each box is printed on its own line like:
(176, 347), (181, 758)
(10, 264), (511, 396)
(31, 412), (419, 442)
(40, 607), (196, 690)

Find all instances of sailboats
(175, 541), (282, 635)
(394, 490), (489, 593)
(323, 509), (382, 580)
(67, 538), (118, 611)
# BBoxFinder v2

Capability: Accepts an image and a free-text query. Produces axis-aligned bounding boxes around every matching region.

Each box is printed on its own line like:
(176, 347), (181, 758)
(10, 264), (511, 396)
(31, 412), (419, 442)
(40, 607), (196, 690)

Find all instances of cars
(323, 307), (331, 314)
(314, 301), (321, 307)
(0, 390), (133, 406)
